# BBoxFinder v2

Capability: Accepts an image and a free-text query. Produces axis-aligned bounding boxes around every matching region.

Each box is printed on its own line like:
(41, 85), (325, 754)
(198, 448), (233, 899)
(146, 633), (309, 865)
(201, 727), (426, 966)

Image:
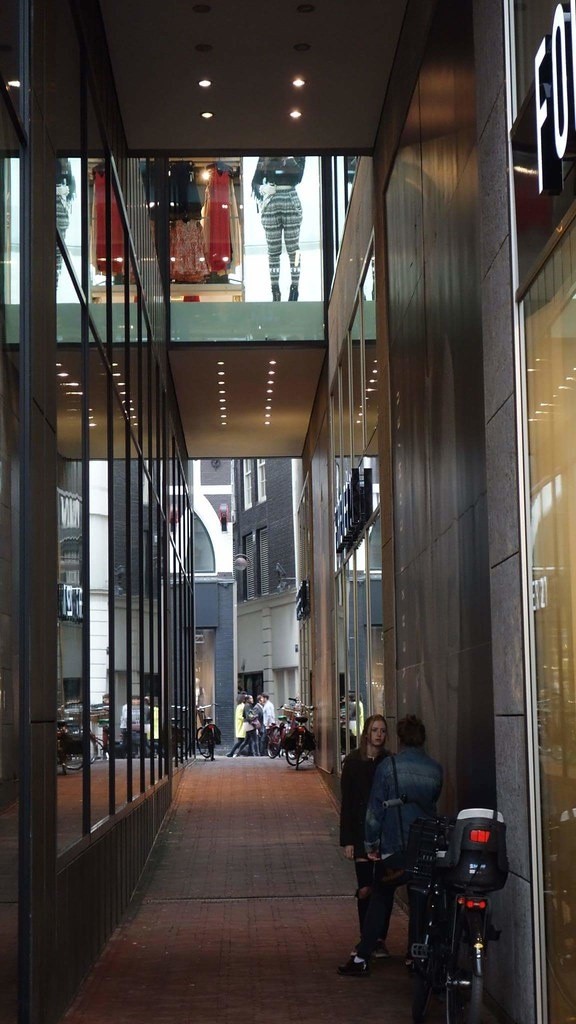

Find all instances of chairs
(435, 808), (509, 893)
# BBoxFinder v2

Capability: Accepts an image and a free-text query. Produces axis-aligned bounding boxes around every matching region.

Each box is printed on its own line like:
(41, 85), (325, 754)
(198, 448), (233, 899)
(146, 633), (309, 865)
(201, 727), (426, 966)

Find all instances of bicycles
(197, 703), (219, 761)
(267, 697), (315, 770)
(381, 792), (511, 1024)
(56, 705), (109, 770)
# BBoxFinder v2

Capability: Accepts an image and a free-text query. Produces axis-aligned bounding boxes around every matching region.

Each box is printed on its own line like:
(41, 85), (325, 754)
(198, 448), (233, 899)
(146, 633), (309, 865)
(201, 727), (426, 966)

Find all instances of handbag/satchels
(281, 726), (318, 751)
(251, 718), (261, 729)
(199, 723), (222, 747)
(374, 851), (413, 886)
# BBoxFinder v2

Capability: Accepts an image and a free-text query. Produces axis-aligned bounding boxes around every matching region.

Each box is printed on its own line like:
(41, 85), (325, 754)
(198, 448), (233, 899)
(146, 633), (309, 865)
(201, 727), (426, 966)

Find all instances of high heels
(272, 283), (281, 302)
(288, 285), (299, 301)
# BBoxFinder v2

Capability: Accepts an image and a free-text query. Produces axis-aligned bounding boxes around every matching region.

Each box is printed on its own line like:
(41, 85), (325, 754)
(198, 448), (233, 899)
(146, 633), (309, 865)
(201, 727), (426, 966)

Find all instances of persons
(56, 158), (76, 294)
(339, 713), (399, 958)
(195, 677), (206, 726)
(250, 156), (305, 301)
(102, 693), (160, 759)
(340, 690), (364, 754)
(227, 691), (276, 758)
(335, 712), (443, 977)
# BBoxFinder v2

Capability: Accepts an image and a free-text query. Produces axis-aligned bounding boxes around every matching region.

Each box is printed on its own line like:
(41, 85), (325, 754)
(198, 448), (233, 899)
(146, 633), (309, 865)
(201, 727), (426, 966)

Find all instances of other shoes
(247, 752), (254, 756)
(227, 753), (233, 757)
(400, 954), (420, 974)
(337, 955), (369, 977)
(236, 752), (240, 757)
(255, 753), (259, 756)
(350, 944), (359, 956)
(260, 751), (265, 756)
(372, 941), (390, 957)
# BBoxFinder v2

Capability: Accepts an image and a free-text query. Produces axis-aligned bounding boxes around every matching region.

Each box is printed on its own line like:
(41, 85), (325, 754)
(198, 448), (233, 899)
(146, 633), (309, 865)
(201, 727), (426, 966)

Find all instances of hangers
(206, 158), (235, 175)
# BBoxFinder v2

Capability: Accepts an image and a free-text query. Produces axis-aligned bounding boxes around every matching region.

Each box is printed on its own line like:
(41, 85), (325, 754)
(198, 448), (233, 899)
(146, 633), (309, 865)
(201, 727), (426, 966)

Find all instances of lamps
(235, 554), (253, 572)
